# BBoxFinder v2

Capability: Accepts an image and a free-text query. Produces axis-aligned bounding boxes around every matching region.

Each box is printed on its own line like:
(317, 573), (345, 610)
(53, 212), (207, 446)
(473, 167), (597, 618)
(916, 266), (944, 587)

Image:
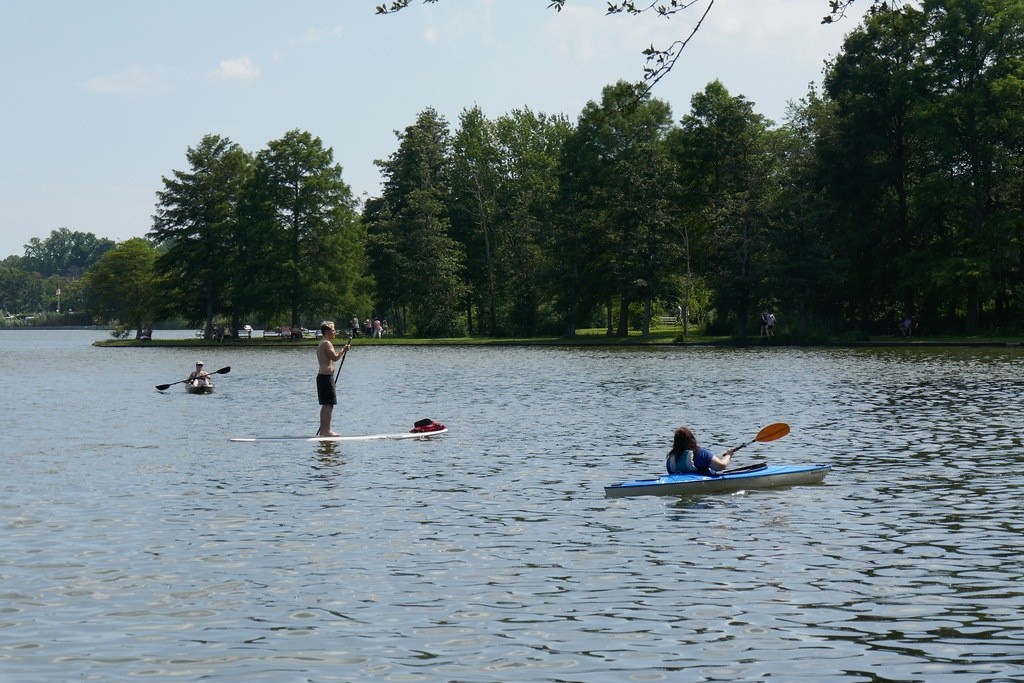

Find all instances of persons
(186, 361), (212, 387)
(141, 327), (151, 340)
(214, 323), (231, 343)
(760, 309), (778, 337)
(278, 323), (301, 341)
(899, 315), (919, 338)
(676, 305), (683, 326)
(350, 314), (388, 339)
(666, 428), (734, 475)
(315, 321), (351, 436)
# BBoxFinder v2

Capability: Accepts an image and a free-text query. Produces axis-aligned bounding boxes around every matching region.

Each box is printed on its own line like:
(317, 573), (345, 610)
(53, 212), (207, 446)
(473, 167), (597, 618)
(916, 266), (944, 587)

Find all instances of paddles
(155, 366), (231, 391)
(723, 423), (791, 457)
(315, 336), (353, 435)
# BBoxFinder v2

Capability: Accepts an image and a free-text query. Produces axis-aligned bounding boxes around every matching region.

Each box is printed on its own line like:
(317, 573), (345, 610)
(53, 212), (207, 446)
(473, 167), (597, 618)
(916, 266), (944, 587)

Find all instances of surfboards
(227, 427), (448, 443)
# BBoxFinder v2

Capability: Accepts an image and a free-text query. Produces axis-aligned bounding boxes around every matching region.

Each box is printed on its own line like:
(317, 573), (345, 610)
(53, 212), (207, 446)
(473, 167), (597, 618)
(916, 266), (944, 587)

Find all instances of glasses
(197, 364), (202, 366)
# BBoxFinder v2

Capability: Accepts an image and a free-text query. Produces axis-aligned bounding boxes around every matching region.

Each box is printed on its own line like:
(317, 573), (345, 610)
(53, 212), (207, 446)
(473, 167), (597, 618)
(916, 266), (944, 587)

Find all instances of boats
(185, 379), (216, 395)
(603, 461), (833, 499)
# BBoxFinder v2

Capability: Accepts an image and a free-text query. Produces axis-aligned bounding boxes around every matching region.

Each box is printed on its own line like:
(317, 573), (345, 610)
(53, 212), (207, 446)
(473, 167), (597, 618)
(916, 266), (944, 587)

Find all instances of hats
(196, 361), (203, 366)
